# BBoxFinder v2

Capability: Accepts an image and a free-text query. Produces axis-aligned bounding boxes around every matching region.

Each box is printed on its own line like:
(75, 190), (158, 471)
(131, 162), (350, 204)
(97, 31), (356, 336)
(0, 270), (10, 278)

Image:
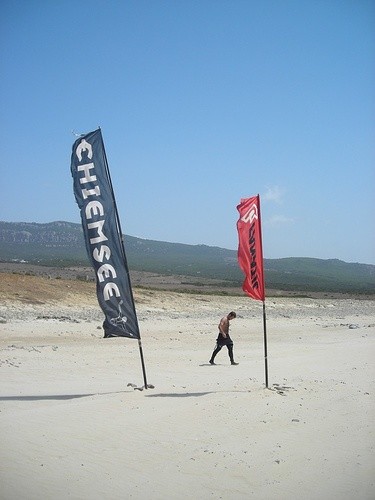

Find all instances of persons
(208, 311), (239, 365)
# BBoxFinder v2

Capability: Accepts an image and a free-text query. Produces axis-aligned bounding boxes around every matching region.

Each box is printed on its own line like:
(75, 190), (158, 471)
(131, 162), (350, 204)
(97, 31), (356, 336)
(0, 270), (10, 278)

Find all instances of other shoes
(231, 361), (239, 365)
(209, 360), (216, 365)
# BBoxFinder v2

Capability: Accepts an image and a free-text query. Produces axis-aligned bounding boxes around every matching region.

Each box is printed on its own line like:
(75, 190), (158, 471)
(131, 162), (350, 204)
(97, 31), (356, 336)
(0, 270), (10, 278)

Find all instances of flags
(70, 129), (141, 339)
(236, 195), (265, 301)
